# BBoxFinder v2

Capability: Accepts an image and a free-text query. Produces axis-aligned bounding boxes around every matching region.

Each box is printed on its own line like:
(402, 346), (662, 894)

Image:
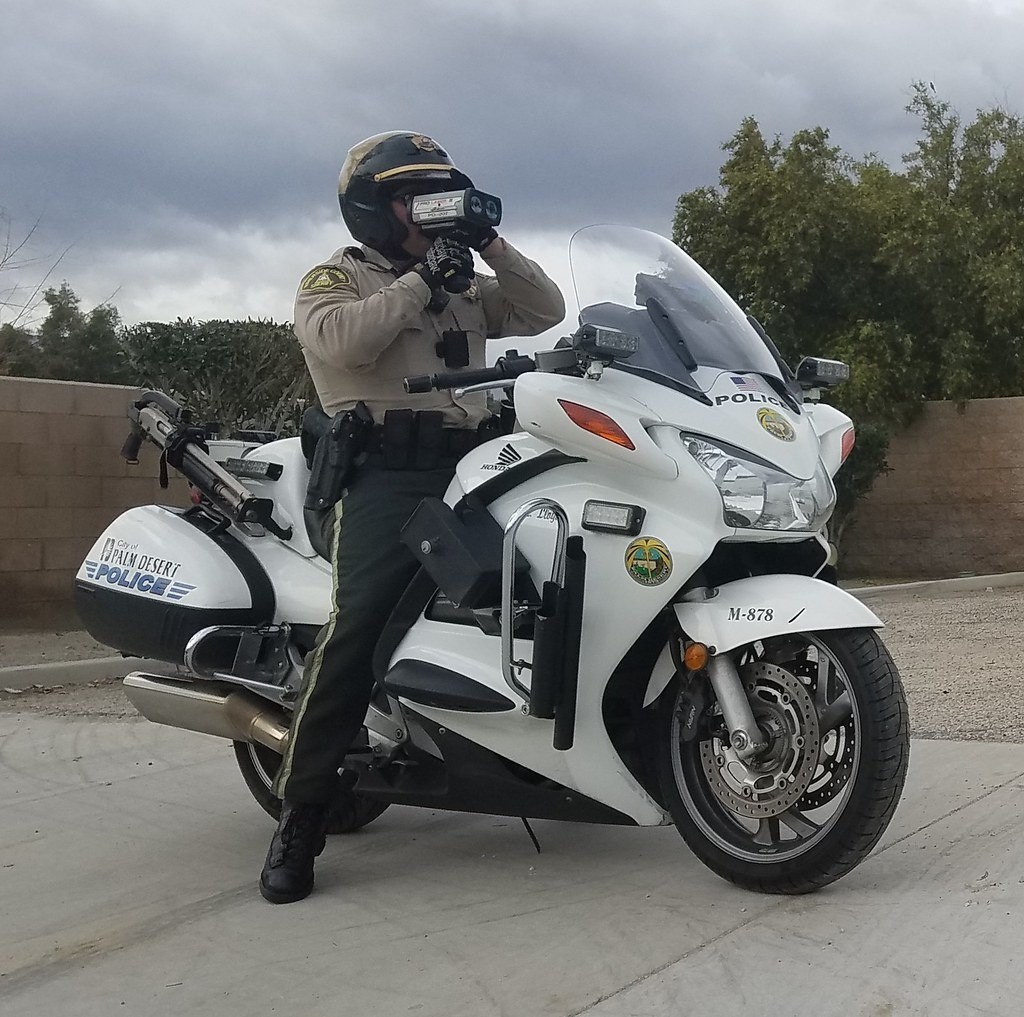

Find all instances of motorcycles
(72, 222), (914, 896)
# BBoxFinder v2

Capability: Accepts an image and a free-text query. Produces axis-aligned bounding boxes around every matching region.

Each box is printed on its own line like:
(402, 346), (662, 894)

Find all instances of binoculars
(407, 188), (501, 294)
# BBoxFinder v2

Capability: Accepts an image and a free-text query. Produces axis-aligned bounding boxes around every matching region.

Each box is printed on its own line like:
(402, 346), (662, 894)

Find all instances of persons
(256, 127), (564, 906)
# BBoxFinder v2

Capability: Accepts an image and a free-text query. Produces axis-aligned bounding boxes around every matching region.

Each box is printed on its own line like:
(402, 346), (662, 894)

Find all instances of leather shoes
(259, 802), (330, 905)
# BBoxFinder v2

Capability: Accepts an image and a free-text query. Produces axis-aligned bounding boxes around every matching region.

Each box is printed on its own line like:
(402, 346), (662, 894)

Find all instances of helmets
(337, 130), (462, 257)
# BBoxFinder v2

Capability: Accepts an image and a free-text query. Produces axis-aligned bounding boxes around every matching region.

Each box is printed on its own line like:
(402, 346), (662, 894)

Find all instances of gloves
(404, 229), (476, 293)
(419, 167), (499, 253)
